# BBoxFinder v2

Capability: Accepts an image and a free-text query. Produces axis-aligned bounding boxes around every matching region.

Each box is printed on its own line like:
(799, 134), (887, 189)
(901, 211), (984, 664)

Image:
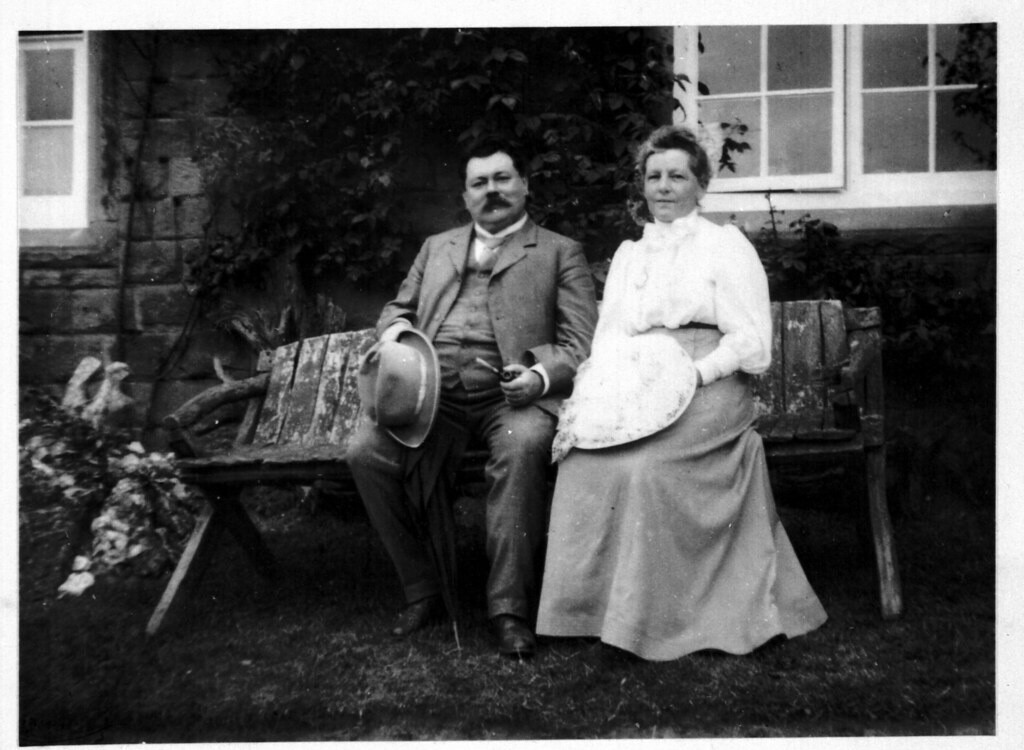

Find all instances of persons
(532, 121), (828, 664)
(342, 132), (599, 659)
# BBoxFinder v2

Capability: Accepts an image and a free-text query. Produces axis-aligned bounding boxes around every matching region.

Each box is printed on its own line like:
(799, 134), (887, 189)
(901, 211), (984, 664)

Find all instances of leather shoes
(390, 593), (448, 639)
(492, 613), (537, 658)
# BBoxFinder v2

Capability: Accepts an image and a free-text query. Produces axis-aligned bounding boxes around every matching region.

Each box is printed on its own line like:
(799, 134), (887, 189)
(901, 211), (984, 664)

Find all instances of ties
(479, 238), (506, 266)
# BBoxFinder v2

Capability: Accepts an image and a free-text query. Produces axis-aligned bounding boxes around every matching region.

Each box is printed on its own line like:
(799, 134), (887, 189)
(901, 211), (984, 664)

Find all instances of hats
(357, 327), (441, 449)
(549, 332), (698, 465)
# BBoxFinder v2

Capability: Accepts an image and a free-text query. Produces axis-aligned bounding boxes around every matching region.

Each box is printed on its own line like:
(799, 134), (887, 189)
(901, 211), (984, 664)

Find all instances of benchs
(141, 299), (905, 647)
